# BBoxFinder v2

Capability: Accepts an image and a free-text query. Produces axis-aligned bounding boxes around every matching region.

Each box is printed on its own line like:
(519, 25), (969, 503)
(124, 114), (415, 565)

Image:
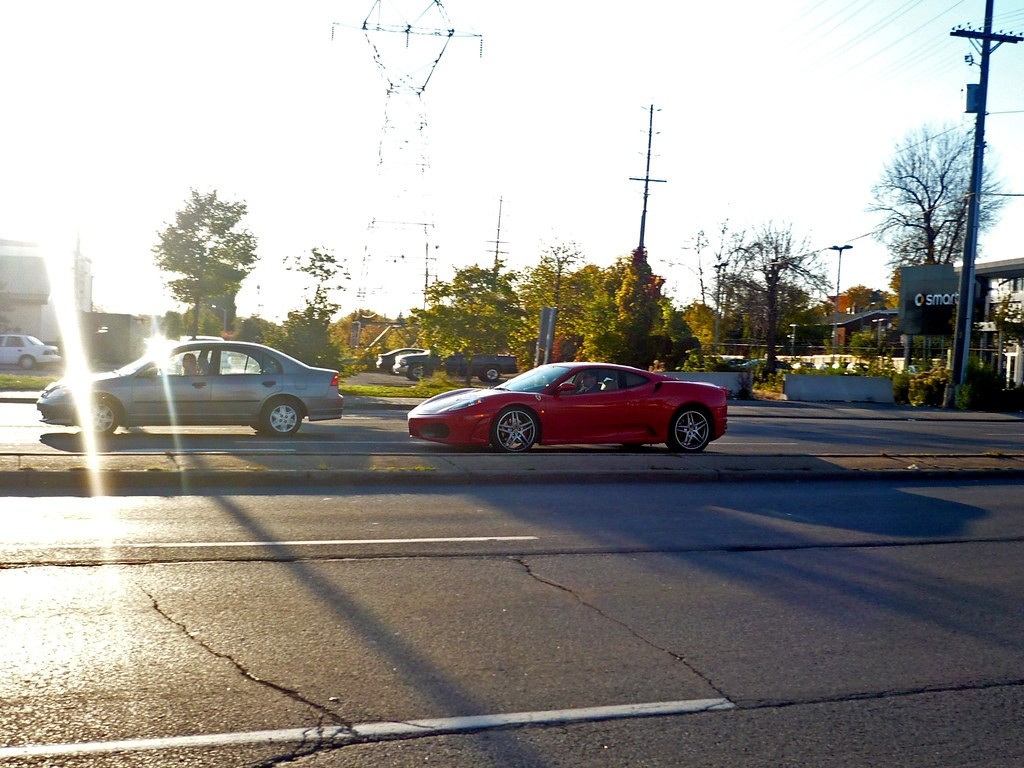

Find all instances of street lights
(829, 244), (854, 355)
(713, 263), (730, 352)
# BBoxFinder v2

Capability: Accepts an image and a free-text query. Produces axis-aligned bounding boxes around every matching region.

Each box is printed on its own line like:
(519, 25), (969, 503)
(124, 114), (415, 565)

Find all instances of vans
(177, 334), (233, 375)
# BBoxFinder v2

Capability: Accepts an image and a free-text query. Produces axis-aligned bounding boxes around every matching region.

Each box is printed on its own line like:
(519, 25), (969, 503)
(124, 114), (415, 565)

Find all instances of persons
(568, 370), (601, 396)
(182, 353), (198, 375)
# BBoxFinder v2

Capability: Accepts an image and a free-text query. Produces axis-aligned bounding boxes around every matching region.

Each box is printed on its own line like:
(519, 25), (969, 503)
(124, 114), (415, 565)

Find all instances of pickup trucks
(392, 351), (519, 382)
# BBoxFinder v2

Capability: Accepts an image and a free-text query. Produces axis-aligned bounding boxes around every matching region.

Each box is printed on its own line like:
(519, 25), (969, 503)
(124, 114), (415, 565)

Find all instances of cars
(0, 333), (61, 370)
(377, 348), (425, 375)
(710, 355), (870, 374)
(35, 340), (342, 436)
(909, 365), (934, 374)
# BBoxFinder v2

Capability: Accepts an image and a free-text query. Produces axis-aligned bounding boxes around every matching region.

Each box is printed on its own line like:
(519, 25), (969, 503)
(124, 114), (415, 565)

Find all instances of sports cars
(409, 361), (729, 455)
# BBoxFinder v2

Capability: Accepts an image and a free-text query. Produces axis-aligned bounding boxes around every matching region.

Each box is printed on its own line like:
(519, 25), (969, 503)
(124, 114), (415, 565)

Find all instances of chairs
(197, 356), (208, 374)
(600, 377), (613, 390)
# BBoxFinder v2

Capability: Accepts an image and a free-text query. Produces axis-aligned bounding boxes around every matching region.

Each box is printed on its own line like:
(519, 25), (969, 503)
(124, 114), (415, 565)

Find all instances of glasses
(582, 376), (594, 379)
